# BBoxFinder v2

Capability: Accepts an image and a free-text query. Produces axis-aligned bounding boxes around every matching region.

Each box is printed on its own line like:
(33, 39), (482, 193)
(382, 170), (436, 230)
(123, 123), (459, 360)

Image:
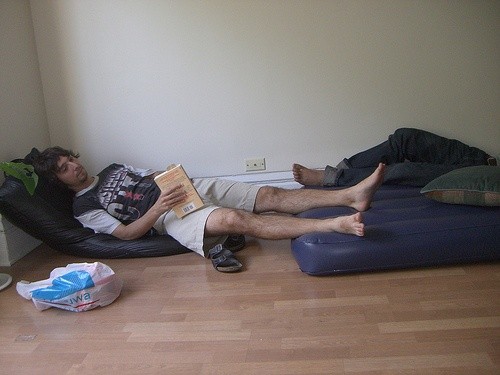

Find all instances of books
(154, 164), (205, 220)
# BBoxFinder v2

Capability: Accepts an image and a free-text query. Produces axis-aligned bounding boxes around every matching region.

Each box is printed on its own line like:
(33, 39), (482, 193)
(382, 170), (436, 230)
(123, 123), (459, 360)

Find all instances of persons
(292, 128), (500, 188)
(34, 146), (386, 260)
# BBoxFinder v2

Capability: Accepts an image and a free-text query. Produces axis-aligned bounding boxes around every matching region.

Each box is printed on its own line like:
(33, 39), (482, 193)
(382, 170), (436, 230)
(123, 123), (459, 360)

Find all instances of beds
(292, 183), (500, 277)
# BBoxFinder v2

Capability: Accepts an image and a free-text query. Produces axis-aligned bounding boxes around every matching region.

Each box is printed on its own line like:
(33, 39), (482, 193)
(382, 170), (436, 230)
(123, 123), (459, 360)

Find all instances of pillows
(420, 166), (500, 207)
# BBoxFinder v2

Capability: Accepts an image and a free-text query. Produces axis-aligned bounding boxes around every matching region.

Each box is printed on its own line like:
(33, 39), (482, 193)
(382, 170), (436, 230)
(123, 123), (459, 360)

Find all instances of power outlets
(246, 158), (265, 171)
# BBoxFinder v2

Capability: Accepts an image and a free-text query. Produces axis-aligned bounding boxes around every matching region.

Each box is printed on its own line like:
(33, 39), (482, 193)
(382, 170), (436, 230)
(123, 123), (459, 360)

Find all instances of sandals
(207, 244), (243, 273)
(225, 234), (245, 251)
